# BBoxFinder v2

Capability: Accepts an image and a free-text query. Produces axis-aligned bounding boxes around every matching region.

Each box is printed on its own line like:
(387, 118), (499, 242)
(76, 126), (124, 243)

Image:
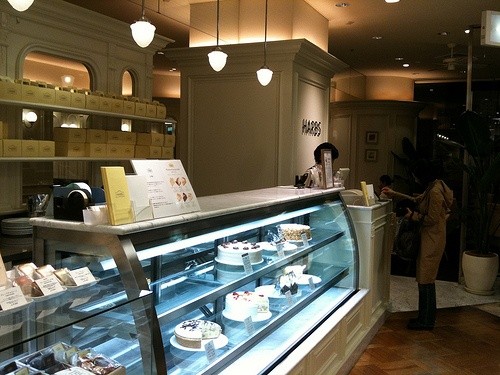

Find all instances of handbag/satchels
(396, 220), (420, 261)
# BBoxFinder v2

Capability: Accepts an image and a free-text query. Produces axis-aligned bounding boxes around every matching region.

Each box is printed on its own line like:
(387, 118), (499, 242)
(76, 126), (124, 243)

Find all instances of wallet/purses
(396, 199), (409, 217)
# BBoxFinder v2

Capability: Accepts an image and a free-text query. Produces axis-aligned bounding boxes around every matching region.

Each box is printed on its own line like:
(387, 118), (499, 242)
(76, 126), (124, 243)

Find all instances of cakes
(173, 320), (222, 349)
(225, 264), (307, 320)
(217, 239), (263, 264)
(280, 224), (311, 240)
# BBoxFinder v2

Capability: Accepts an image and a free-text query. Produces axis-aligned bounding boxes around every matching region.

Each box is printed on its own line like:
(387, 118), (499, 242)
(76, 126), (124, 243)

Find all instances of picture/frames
(365, 131), (379, 145)
(364, 149), (377, 162)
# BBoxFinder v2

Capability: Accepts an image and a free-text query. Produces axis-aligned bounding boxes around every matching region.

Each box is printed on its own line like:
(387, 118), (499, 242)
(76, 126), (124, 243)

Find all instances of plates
(1, 217), (33, 236)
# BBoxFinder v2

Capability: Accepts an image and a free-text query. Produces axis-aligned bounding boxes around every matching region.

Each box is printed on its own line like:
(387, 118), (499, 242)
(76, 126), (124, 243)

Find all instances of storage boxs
(0, 76), (175, 160)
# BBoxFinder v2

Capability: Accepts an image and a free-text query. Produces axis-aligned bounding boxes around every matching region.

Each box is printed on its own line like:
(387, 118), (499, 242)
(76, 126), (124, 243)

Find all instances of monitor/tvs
(335, 168), (350, 179)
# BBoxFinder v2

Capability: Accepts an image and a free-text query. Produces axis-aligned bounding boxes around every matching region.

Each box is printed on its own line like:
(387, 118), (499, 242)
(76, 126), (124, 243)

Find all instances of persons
(379, 159), (453, 331)
(304, 142), (339, 187)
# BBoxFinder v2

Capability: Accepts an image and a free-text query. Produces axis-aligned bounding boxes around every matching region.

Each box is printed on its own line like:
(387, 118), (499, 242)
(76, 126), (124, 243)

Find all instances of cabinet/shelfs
(0, 99), (178, 161)
(29, 186), (361, 375)
(0, 284), (153, 375)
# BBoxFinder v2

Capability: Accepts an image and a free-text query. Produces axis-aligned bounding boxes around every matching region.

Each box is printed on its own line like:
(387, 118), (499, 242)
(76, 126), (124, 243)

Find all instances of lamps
(256, 0), (273, 86)
(207, 0), (228, 72)
(130, 0), (156, 48)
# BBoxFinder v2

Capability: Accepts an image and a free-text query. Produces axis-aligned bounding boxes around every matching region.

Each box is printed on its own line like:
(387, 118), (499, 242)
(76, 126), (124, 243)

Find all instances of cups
(82, 203), (109, 226)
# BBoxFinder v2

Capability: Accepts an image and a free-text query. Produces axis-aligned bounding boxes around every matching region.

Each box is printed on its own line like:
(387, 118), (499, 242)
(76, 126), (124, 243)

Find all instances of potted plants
(433, 110), (500, 296)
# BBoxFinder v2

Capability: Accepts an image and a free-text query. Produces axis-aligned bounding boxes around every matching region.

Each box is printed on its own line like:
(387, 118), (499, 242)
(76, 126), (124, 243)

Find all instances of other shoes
(406, 318), (434, 331)
